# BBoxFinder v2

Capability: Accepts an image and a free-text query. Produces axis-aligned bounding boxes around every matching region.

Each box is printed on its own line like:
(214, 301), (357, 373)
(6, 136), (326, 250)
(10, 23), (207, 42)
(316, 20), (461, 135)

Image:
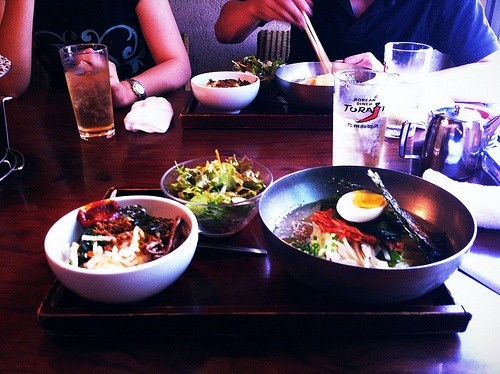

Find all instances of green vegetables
(231, 55), (285, 80)
(376, 243), (403, 268)
(169, 149), (267, 235)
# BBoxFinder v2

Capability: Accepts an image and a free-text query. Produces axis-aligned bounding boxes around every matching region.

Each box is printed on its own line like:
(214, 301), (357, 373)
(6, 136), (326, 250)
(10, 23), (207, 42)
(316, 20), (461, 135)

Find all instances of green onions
(287, 234), (344, 255)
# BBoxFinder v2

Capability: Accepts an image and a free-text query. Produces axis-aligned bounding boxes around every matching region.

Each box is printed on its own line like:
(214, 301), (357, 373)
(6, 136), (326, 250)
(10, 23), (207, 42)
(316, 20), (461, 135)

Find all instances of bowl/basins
(191, 71), (261, 114)
(44, 194), (200, 304)
(274, 61), (376, 110)
(258, 165), (478, 302)
(160, 156), (275, 236)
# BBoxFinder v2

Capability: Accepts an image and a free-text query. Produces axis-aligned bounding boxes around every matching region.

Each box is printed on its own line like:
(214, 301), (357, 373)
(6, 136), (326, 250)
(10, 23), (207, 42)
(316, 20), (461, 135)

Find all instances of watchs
(126, 77), (147, 100)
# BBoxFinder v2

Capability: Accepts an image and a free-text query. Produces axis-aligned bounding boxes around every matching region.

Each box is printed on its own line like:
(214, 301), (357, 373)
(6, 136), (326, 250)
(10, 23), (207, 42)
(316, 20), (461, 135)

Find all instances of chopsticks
(301, 10), (333, 74)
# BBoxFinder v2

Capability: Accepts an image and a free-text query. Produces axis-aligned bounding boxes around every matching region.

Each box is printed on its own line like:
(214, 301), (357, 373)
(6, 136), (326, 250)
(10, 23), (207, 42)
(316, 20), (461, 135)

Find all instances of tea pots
(399, 105), (500, 181)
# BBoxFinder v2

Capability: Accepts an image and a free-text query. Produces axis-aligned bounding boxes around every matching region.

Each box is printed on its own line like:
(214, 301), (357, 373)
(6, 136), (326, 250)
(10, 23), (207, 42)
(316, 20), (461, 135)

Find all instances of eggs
(336, 190), (387, 223)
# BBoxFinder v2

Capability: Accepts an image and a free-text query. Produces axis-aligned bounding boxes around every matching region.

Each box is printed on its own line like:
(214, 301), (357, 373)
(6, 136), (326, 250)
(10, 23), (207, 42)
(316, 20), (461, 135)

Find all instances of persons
(214, 0), (500, 112)
(0, 0), (192, 108)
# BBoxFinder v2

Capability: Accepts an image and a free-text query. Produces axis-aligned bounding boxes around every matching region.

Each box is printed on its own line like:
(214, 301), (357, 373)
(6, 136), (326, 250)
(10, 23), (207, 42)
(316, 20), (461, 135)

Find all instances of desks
(0, 89), (500, 374)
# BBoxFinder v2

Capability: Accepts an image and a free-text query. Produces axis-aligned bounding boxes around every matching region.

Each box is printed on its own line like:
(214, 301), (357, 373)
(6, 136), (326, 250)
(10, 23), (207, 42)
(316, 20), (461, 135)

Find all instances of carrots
(309, 208), (405, 248)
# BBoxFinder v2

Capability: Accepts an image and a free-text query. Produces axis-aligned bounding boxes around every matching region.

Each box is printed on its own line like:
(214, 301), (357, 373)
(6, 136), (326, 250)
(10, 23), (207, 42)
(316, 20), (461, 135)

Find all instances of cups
(332, 69), (399, 168)
(383, 41), (439, 139)
(59, 44), (116, 140)
